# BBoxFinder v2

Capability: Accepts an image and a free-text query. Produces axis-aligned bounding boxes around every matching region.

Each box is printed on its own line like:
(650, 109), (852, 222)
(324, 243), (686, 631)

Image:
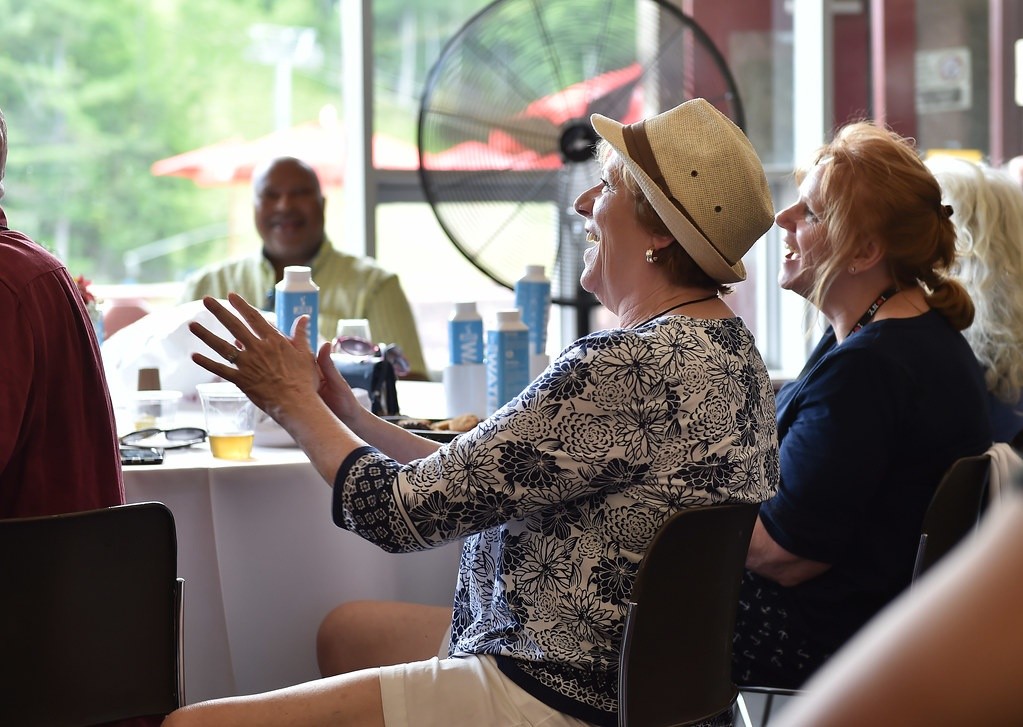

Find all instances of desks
(122, 415), (465, 707)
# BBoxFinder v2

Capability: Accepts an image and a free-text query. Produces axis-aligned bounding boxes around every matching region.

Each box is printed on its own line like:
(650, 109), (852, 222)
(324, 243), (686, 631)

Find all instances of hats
(589, 94), (777, 285)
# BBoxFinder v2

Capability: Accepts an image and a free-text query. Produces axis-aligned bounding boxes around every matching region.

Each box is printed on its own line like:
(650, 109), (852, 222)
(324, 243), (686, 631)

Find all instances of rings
(225, 348), (240, 364)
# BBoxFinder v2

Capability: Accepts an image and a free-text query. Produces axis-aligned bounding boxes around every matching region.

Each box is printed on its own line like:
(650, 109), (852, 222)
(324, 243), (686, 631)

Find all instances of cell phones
(118, 448), (164, 466)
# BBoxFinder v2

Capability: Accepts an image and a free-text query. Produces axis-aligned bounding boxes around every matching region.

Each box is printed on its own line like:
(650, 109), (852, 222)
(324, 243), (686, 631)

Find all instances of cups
(196, 381), (256, 459)
(529, 356), (549, 384)
(443, 364), (489, 421)
(337, 318), (372, 346)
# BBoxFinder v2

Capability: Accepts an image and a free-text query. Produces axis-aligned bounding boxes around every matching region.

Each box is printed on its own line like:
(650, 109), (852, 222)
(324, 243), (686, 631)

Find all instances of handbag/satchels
(329, 338), (399, 426)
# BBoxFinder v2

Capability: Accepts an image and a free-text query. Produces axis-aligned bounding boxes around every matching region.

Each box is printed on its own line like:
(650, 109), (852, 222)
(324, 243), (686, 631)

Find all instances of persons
(176, 156), (430, 383)
(734, 120), (1023, 727)
(158, 97), (776, 727)
(0, 111), (128, 522)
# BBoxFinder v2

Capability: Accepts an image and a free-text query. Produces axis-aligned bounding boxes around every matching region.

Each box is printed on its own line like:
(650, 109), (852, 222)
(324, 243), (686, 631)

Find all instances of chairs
(736, 429), (1023, 727)
(0, 501), (187, 727)
(617, 502), (762, 727)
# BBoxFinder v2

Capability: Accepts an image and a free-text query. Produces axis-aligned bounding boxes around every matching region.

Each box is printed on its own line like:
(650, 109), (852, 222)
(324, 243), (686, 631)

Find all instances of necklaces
(631, 293), (719, 330)
(833, 276), (919, 340)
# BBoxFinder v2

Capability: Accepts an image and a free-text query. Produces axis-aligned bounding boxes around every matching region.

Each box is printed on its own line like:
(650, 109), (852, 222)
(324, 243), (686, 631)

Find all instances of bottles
(448, 302), (484, 365)
(514, 266), (551, 356)
(275, 265), (318, 358)
(487, 310), (529, 414)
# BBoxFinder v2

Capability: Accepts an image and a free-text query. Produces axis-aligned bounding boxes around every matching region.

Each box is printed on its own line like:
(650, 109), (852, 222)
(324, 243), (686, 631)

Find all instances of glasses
(120, 428), (206, 450)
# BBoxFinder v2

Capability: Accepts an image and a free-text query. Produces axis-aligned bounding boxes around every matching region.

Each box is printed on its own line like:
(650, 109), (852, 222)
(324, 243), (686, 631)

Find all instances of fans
(417, 1), (747, 341)
